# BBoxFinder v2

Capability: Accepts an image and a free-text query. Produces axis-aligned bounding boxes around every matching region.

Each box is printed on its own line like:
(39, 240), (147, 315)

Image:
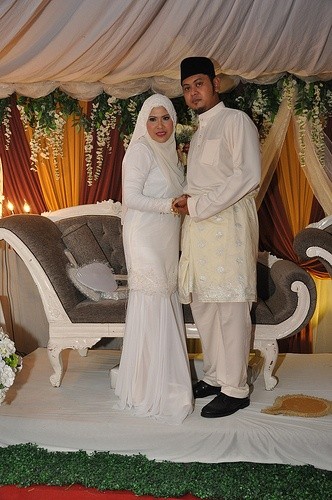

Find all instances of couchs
(0, 199), (318, 391)
(293, 214), (332, 281)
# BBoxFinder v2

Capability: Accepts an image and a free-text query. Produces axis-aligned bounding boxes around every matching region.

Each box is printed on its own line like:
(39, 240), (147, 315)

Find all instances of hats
(130, 93), (186, 197)
(180, 57), (215, 86)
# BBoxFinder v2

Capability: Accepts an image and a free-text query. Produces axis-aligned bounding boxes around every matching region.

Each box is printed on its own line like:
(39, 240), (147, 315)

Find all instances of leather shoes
(201, 393), (250, 418)
(192, 380), (222, 398)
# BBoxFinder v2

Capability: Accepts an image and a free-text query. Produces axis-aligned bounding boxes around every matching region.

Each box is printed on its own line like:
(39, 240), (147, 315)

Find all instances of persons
(172, 56), (261, 418)
(108, 93), (195, 423)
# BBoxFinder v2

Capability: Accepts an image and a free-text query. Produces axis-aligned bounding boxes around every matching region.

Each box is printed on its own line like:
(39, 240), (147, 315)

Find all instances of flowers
(0, 327), (23, 404)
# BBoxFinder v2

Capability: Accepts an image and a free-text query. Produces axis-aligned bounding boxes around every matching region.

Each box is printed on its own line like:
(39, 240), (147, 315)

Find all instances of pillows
(59, 221), (111, 268)
